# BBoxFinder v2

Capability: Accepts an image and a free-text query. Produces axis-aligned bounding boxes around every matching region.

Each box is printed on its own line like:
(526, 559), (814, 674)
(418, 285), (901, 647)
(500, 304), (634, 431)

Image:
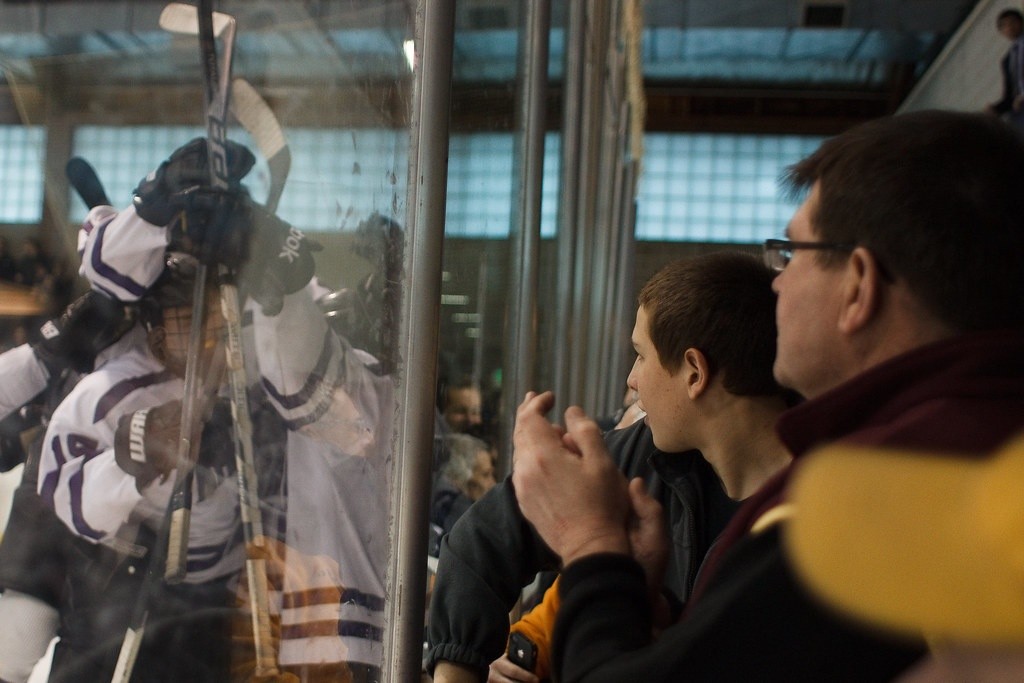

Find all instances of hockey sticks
(157, 0), (238, 587)
(66, 157), (111, 214)
(107, 74), (297, 683)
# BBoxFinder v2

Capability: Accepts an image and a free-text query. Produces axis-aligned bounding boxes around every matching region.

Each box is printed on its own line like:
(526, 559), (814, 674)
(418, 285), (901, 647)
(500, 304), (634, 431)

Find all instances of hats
(785, 436), (1024, 648)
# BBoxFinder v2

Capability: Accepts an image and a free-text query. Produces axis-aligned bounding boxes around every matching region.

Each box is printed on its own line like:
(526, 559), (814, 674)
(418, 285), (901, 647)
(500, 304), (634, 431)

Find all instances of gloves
(114, 397), (232, 490)
(132, 137), (256, 227)
(35, 292), (126, 374)
(185, 185), (324, 315)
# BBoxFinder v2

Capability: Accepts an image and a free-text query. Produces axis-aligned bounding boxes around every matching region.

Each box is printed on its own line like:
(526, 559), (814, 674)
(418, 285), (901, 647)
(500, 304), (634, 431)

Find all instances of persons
(0, 137), (408, 683)
(422, 7), (1024, 683)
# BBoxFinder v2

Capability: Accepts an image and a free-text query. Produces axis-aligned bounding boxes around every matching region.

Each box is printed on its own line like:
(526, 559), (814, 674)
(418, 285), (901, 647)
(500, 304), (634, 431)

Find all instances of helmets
(314, 279), (353, 333)
(138, 250), (229, 376)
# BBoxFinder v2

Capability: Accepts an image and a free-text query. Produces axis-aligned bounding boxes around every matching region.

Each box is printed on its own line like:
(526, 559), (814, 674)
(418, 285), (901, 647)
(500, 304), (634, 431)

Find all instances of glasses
(764, 239), (896, 287)
